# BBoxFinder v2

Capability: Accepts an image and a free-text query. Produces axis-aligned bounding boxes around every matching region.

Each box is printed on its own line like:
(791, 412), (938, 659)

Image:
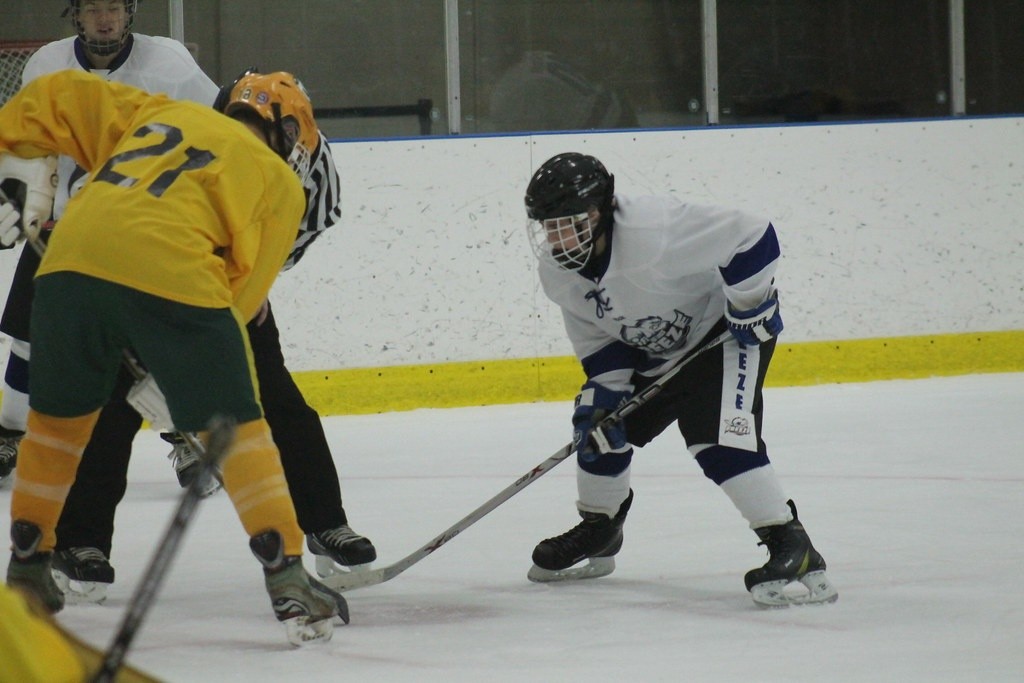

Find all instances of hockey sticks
(90, 432), (225, 683)
(311, 325), (731, 592)
(0, 189), (226, 501)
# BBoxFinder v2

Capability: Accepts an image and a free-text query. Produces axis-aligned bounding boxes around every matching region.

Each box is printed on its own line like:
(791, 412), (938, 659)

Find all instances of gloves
(571, 380), (635, 462)
(724, 296), (783, 350)
(0, 178), (27, 250)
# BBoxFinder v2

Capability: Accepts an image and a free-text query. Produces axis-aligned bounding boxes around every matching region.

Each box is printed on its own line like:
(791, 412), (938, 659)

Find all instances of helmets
(61, 0), (138, 55)
(213, 68), (259, 113)
(223, 71), (319, 187)
(524, 152), (614, 272)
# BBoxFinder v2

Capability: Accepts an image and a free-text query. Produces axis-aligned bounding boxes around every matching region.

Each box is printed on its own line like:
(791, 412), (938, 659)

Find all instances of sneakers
(50, 546), (115, 604)
(744, 499), (838, 609)
(0, 424), (26, 488)
(250, 529), (339, 647)
(527, 488), (634, 583)
(160, 432), (223, 498)
(6, 519), (66, 618)
(303, 521), (376, 579)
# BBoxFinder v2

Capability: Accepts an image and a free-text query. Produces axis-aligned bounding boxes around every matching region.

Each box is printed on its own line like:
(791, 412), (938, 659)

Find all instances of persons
(523, 151), (839, 611)
(0, 1), (378, 683)
